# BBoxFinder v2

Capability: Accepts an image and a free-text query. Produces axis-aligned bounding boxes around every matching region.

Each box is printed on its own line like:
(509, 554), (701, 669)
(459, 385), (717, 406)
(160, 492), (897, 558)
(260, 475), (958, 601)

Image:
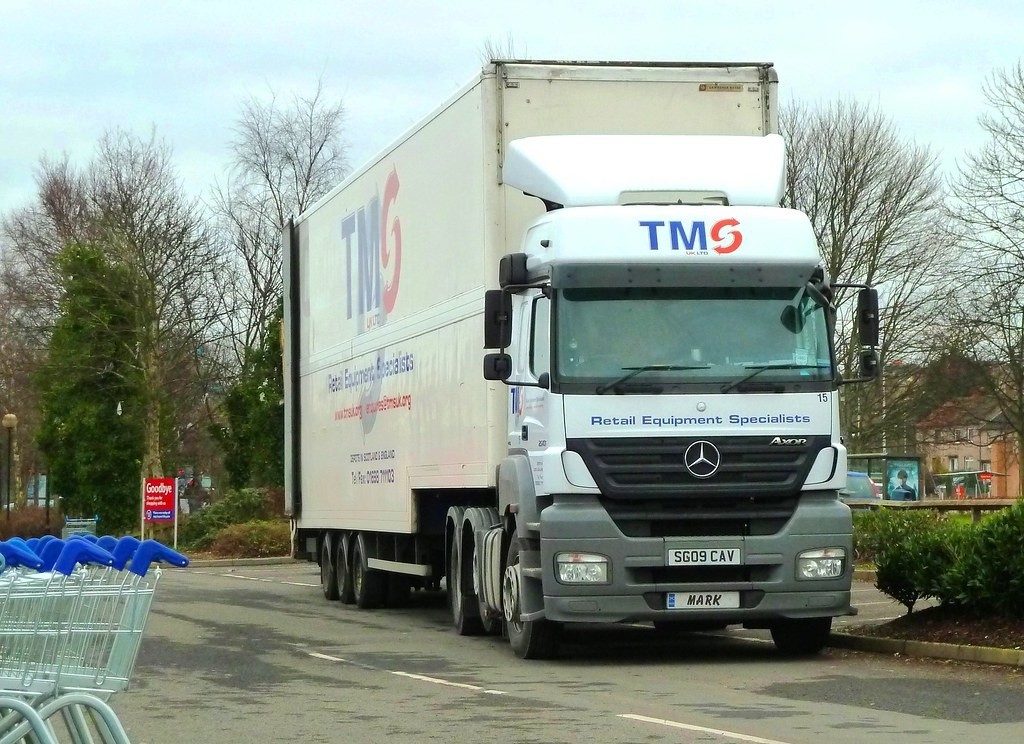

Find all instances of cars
(839, 472), (881, 500)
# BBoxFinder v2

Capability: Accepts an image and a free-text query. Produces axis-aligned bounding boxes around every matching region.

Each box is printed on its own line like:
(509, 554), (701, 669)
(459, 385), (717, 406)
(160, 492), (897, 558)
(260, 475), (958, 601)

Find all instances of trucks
(280, 57), (879, 660)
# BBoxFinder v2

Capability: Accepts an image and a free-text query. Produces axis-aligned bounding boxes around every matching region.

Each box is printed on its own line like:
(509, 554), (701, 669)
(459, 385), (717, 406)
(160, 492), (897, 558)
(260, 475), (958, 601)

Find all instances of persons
(892, 471), (916, 501)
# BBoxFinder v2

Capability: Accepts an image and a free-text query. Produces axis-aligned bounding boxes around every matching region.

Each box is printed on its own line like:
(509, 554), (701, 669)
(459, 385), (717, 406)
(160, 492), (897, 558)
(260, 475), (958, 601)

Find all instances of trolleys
(0, 532), (189, 744)
(62, 515), (98, 540)
